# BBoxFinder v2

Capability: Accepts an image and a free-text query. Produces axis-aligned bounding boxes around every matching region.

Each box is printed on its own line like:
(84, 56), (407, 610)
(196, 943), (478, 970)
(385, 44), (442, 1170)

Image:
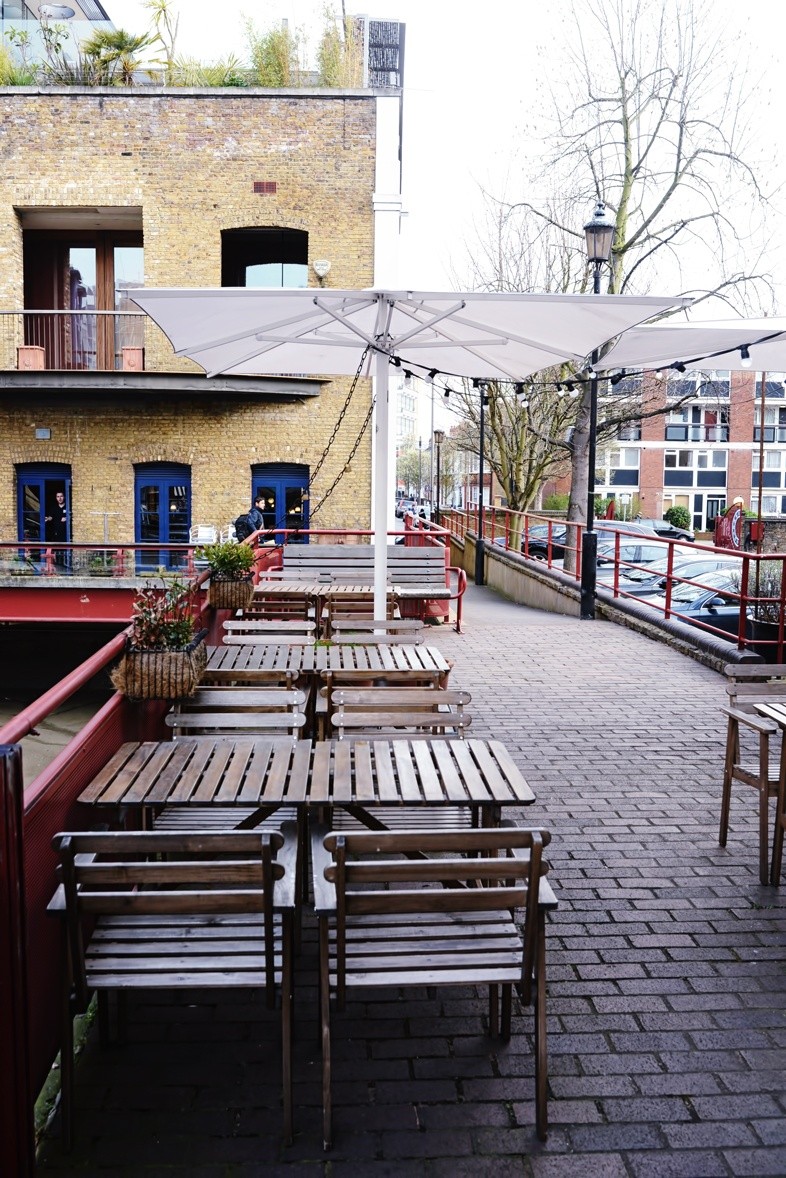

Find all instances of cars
(540, 538), (685, 577)
(395, 500), (434, 528)
(590, 554), (786, 648)
(488, 519), (660, 563)
(629, 518), (696, 542)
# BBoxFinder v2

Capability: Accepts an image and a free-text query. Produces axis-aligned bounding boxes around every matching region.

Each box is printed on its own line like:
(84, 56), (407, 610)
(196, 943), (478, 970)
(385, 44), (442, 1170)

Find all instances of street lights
(433, 430), (445, 542)
(475, 379), (489, 584)
(581, 200), (617, 623)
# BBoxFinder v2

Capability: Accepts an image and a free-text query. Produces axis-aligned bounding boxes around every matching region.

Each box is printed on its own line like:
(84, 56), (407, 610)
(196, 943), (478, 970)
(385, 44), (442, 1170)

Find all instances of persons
(46, 492), (67, 542)
(418, 508), (430, 530)
(235, 495), (266, 547)
(409, 504), (416, 515)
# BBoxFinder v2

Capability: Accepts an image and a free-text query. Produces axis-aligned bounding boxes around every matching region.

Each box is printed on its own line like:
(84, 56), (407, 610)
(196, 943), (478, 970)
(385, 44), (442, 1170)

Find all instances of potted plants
(88, 555), (116, 576)
(194, 542), (255, 607)
(110, 571), (208, 699)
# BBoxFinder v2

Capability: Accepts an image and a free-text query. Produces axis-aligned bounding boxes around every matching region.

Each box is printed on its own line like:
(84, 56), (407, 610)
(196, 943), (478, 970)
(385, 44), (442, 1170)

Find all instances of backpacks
(235, 508), (258, 543)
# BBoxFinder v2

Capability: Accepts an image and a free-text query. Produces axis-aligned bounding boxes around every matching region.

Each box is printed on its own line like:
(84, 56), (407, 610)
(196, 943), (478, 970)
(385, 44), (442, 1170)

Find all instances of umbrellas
(591, 319), (786, 375)
(113, 288), (693, 644)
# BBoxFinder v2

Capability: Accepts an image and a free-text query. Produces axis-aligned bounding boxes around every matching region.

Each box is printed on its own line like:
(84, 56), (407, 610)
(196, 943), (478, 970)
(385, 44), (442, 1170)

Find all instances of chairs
(715, 664), (786, 886)
(188, 523), (239, 572)
(46, 571), (559, 1155)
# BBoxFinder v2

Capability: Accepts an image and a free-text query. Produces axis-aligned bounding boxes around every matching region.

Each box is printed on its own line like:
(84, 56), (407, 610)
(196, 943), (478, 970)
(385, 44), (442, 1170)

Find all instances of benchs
(258, 544), (451, 619)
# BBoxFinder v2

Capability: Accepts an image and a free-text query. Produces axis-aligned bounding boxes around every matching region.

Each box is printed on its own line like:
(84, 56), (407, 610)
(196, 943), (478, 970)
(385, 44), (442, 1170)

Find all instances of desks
(202, 645), (450, 735)
(252, 583), (399, 620)
(75, 740), (535, 1038)
